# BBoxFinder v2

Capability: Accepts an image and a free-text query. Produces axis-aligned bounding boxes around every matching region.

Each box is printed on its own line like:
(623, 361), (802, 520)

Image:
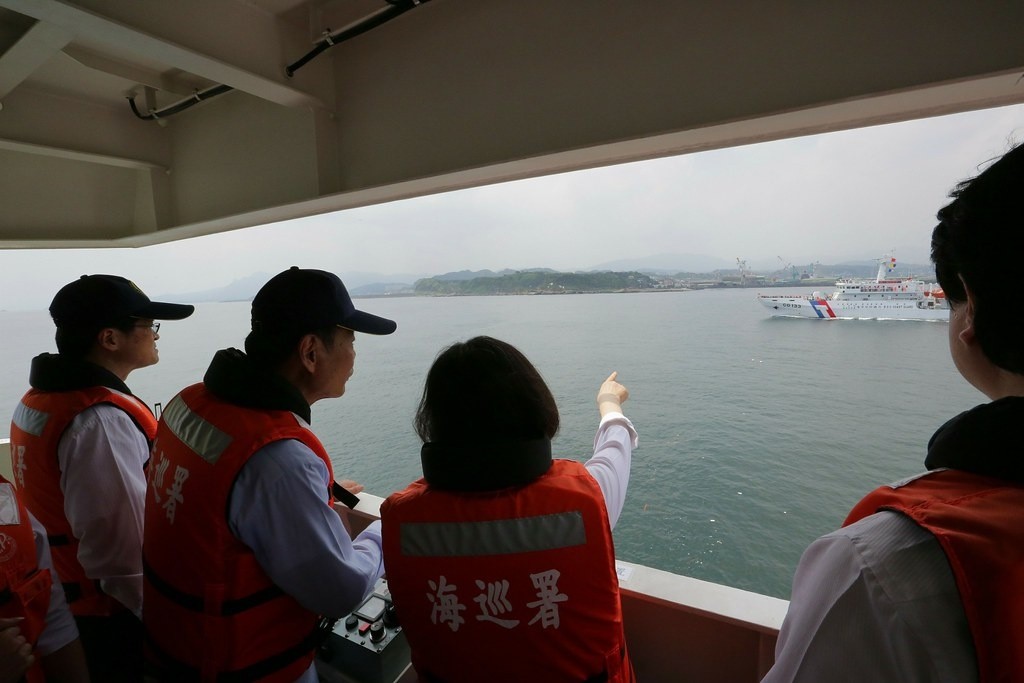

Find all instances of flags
(888, 258), (897, 273)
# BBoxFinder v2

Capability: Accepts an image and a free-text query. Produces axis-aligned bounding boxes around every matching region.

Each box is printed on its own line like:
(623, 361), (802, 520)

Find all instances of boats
(755, 252), (951, 321)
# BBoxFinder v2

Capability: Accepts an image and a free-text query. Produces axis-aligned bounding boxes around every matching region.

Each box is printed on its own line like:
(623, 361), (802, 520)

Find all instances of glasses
(134, 322), (160, 334)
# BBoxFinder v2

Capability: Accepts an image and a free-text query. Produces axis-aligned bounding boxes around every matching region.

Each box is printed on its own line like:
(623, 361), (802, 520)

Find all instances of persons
(378, 334), (641, 683)
(0, 472), (88, 682)
(9, 274), (161, 683)
(762, 139), (1024, 683)
(138, 264), (389, 683)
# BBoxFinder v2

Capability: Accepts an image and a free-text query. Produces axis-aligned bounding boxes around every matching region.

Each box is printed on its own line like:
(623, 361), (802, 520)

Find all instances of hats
(251, 266), (397, 335)
(49, 275), (194, 327)
